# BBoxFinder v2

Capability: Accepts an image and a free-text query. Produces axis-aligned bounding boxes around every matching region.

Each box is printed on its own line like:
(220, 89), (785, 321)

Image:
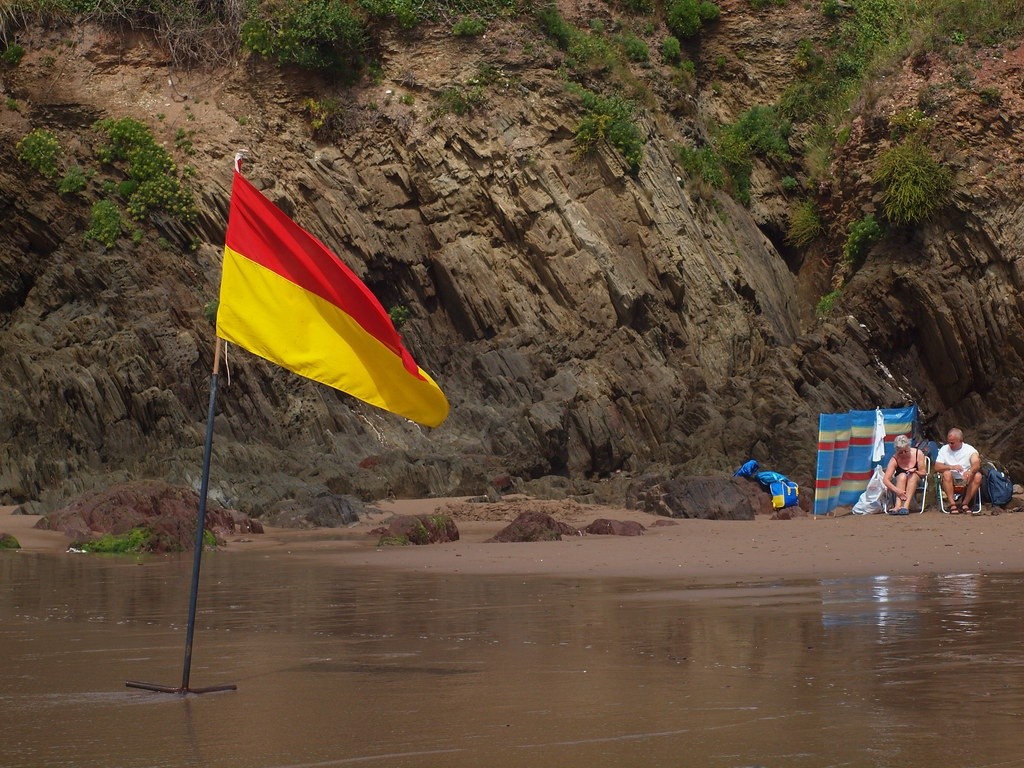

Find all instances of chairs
(884, 456), (931, 514)
(936, 470), (982, 514)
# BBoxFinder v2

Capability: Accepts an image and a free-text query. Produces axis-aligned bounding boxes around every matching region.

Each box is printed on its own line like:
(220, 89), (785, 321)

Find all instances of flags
(216, 155), (450, 428)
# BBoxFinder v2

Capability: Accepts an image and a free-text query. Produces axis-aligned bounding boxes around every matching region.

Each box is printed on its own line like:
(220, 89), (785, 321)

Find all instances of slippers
(889, 507), (909, 515)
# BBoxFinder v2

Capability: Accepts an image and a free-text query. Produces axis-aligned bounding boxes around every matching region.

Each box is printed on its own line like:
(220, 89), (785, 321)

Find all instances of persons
(882, 434), (926, 515)
(934, 428), (983, 515)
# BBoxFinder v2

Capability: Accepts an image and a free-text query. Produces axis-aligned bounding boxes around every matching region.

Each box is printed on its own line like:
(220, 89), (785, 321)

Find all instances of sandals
(950, 504), (959, 514)
(961, 504), (973, 513)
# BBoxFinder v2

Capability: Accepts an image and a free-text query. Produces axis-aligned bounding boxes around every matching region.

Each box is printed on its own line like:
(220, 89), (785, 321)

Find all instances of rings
(967, 475), (968, 477)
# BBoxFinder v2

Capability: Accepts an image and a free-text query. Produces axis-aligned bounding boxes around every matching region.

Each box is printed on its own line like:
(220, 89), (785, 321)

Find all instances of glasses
(898, 448), (909, 454)
(946, 441), (956, 444)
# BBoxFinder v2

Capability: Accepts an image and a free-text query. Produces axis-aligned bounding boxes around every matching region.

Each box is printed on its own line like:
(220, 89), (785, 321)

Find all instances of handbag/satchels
(755, 468), (787, 493)
(851, 464), (895, 516)
(981, 474), (992, 504)
(733, 459), (760, 479)
(769, 480), (799, 510)
(987, 468), (1013, 506)
